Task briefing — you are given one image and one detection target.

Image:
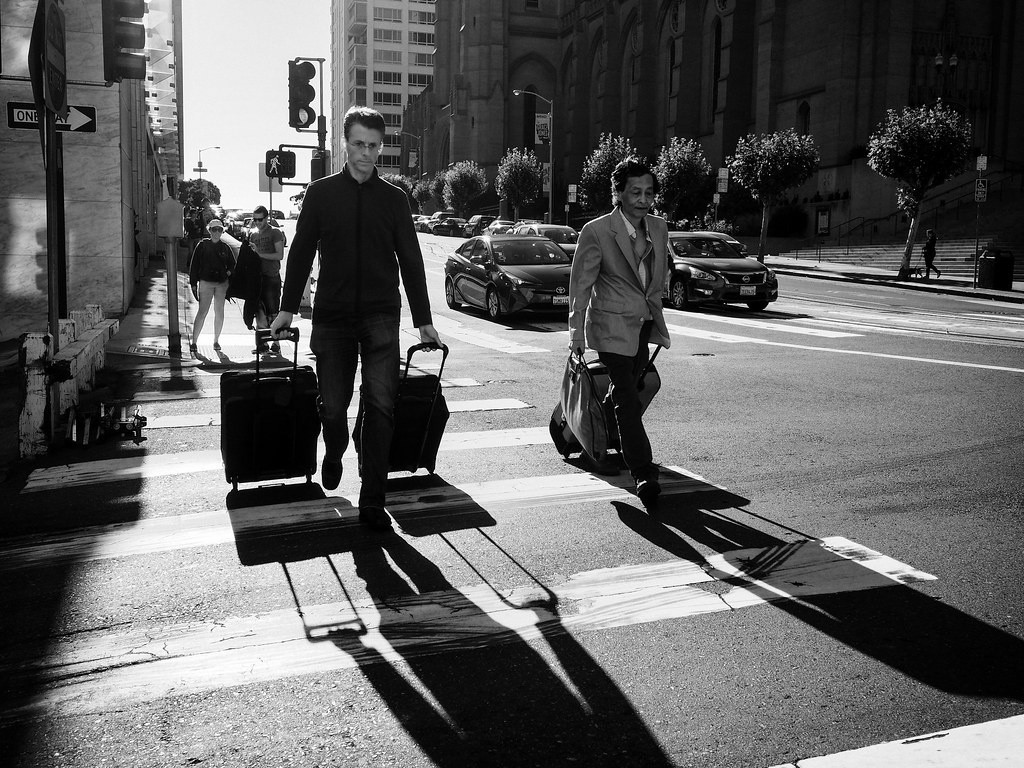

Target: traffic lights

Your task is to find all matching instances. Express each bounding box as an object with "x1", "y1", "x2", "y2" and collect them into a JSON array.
[
  {"x1": 101, "y1": 1, "x2": 147, "y2": 84},
  {"x1": 288, "y1": 60, "x2": 316, "y2": 129},
  {"x1": 265, "y1": 149, "x2": 296, "y2": 178}
]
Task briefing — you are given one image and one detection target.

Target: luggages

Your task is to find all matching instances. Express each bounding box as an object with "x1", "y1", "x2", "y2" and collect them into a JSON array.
[
  {"x1": 352, "y1": 341, "x2": 450, "y2": 474},
  {"x1": 550, "y1": 341, "x2": 662, "y2": 462},
  {"x1": 221, "y1": 327, "x2": 321, "y2": 490}
]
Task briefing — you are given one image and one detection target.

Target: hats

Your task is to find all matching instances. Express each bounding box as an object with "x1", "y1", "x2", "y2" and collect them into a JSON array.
[{"x1": 206, "y1": 219, "x2": 224, "y2": 234}]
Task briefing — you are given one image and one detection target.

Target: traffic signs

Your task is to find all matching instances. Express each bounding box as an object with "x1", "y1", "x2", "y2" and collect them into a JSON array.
[{"x1": 6, "y1": 101, "x2": 99, "y2": 133}]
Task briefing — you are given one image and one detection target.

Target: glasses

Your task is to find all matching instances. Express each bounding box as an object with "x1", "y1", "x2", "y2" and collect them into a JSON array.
[
  {"x1": 208, "y1": 227, "x2": 223, "y2": 232},
  {"x1": 253, "y1": 215, "x2": 266, "y2": 223},
  {"x1": 347, "y1": 141, "x2": 382, "y2": 153}
]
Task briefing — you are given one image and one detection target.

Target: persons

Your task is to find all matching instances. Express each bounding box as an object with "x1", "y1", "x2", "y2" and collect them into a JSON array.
[
  {"x1": 921, "y1": 229, "x2": 941, "y2": 279},
  {"x1": 189, "y1": 219, "x2": 236, "y2": 352},
  {"x1": 569, "y1": 161, "x2": 670, "y2": 498},
  {"x1": 270, "y1": 107, "x2": 444, "y2": 529},
  {"x1": 248, "y1": 206, "x2": 284, "y2": 354}
]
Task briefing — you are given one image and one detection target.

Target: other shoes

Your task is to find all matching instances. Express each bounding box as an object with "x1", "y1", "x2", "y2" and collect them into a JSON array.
[
  {"x1": 322, "y1": 452, "x2": 343, "y2": 490},
  {"x1": 923, "y1": 276, "x2": 929, "y2": 279},
  {"x1": 579, "y1": 451, "x2": 620, "y2": 475},
  {"x1": 635, "y1": 475, "x2": 662, "y2": 498},
  {"x1": 937, "y1": 270, "x2": 941, "y2": 278},
  {"x1": 269, "y1": 343, "x2": 281, "y2": 354},
  {"x1": 359, "y1": 507, "x2": 392, "y2": 527},
  {"x1": 213, "y1": 342, "x2": 220, "y2": 350},
  {"x1": 251, "y1": 344, "x2": 268, "y2": 354},
  {"x1": 190, "y1": 343, "x2": 197, "y2": 351}
]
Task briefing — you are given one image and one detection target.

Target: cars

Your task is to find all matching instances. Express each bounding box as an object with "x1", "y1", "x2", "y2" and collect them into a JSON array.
[
  {"x1": 211, "y1": 204, "x2": 318, "y2": 308},
  {"x1": 512, "y1": 224, "x2": 580, "y2": 261},
  {"x1": 444, "y1": 233, "x2": 573, "y2": 323},
  {"x1": 667, "y1": 231, "x2": 778, "y2": 313},
  {"x1": 412, "y1": 211, "x2": 544, "y2": 239}
]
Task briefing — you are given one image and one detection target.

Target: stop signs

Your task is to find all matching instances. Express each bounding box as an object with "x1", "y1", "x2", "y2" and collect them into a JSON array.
[{"x1": 45, "y1": 4, "x2": 67, "y2": 113}]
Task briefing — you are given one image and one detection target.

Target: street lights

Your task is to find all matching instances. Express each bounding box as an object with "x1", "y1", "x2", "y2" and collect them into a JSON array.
[
  {"x1": 199, "y1": 146, "x2": 221, "y2": 179},
  {"x1": 513, "y1": 90, "x2": 552, "y2": 225},
  {"x1": 394, "y1": 131, "x2": 422, "y2": 183}
]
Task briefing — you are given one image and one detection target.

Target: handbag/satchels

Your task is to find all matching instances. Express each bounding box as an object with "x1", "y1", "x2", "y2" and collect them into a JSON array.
[{"x1": 560, "y1": 342, "x2": 608, "y2": 462}]
